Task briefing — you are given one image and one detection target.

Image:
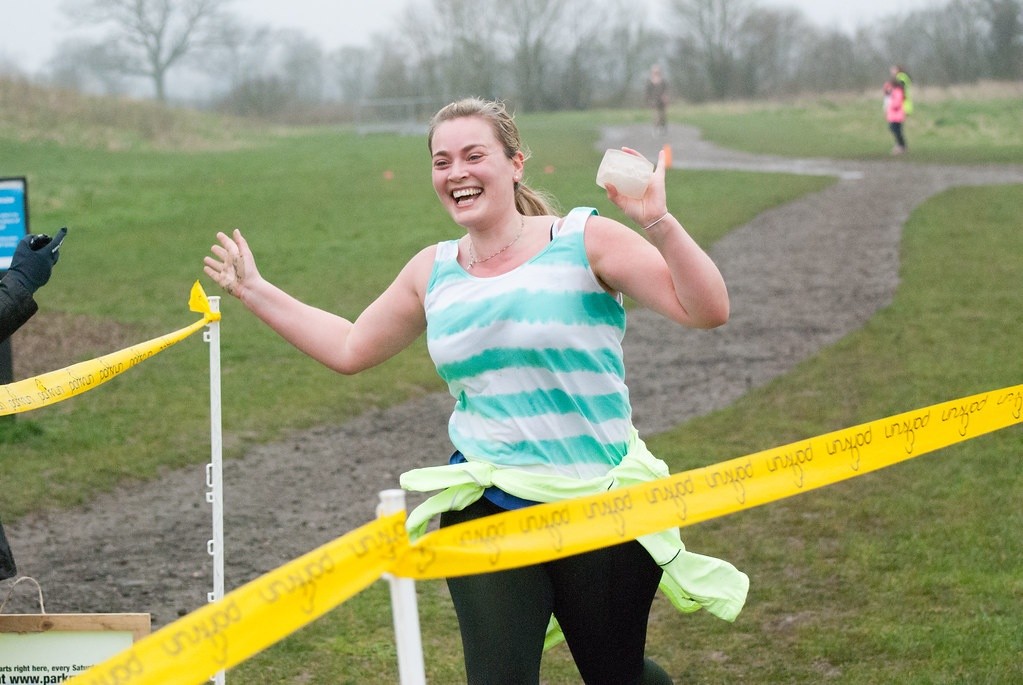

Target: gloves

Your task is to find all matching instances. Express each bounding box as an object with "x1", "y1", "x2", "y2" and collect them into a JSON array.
[{"x1": 5, "y1": 227, "x2": 68, "y2": 295}]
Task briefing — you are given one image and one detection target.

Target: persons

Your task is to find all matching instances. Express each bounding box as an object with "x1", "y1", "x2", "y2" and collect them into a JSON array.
[
  {"x1": 884, "y1": 64, "x2": 913, "y2": 156},
  {"x1": 645, "y1": 62, "x2": 669, "y2": 127},
  {"x1": 202, "y1": 94, "x2": 730, "y2": 685},
  {"x1": 0, "y1": 228, "x2": 68, "y2": 585}
]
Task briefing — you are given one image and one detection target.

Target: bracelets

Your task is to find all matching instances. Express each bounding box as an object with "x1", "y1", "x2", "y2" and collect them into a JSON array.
[{"x1": 643, "y1": 212, "x2": 669, "y2": 234}]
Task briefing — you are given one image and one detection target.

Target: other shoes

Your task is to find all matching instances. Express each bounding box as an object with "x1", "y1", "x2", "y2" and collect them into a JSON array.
[{"x1": 891, "y1": 146, "x2": 904, "y2": 156}]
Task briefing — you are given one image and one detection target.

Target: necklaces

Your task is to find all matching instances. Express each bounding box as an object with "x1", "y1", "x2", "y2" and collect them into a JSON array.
[{"x1": 467, "y1": 214, "x2": 526, "y2": 271}]
